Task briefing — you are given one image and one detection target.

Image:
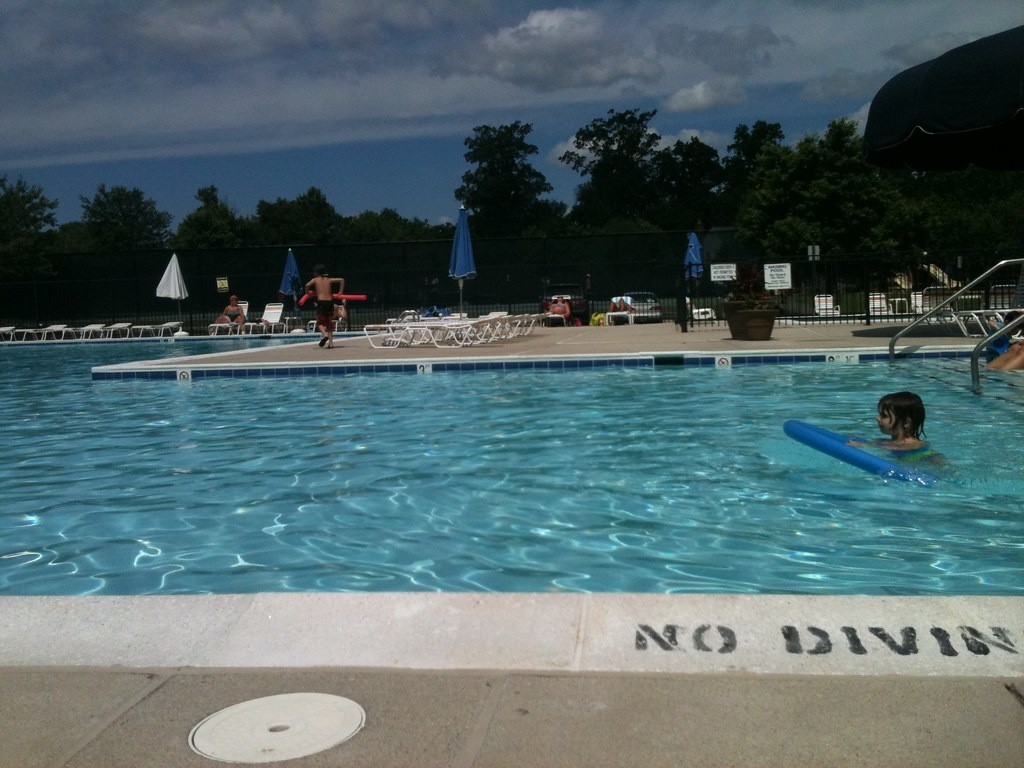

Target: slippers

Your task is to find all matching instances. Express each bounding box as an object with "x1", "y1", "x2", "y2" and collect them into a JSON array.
[
  {"x1": 327, "y1": 344, "x2": 334, "y2": 348},
  {"x1": 319, "y1": 337, "x2": 329, "y2": 347}
]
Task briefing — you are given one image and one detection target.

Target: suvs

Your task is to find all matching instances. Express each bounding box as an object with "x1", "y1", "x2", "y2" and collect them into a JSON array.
[{"x1": 541, "y1": 279, "x2": 591, "y2": 326}]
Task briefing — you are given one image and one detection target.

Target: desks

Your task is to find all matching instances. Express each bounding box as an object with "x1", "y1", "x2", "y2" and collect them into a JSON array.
[{"x1": 284, "y1": 317, "x2": 304, "y2": 333}]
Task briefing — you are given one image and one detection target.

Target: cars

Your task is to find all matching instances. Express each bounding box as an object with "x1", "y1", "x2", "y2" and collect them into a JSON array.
[{"x1": 619, "y1": 291, "x2": 665, "y2": 322}]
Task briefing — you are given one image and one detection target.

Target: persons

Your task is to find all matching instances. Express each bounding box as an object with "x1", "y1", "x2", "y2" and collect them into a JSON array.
[
  {"x1": 544, "y1": 296, "x2": 571, "y2": 317},
  {"x1": 305, "y1": 264, "x2": 348, "y2": 349},
  {"x1": 847, "y1": 391, "x2": 948, "y2": 469},
  {"x1": 984, "y1": 310, "x2": 1024, "y2": 371},
  {"x1": 608, "y1": 296, "x2": 636, "y2": 312},
  {"x1": 215, "y1": 294, "x2": 245, "y2": 335}
]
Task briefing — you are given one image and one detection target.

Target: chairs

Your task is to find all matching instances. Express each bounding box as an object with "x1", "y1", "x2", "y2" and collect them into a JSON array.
[
  {"x1": 911, "y1": 286, "x2": 953, "y2": 314},
  {"x1": 605, "y1": 296, "x2": 634, "y2": 325},
  {"x1": 0, "y1": 322, "x2": 183, "y2": 342},
  {"x1": 307, "y1": 300, "x2": 347, "y2": 333},
  {"x1": 911, "y1": 308, "x2": 1024, "y2": 338},
  {"x1": 989, "y1": 284, "x2": 1017, "y2": 310},
  {"x1": 813, "y1": 294, "x2": 840, "y2": 316},
  {"x1": 869, "y1": 292, "x2": 892, "y2": 314},
  {"x1": 684, "y1": 297, "x2": 717, "y2": 320},
  {"x1": 242, "y1": 302, "x2": 286, "y2": 335},
  {"x1": 542, "y1": 295, "x2": 571, "y2": 327},
  {"x1": 209, "y1": 301, "x2": 248, "y2": 336},
  {"x1": 364, "y1": 311, "x2": 548, "y2": 349}
]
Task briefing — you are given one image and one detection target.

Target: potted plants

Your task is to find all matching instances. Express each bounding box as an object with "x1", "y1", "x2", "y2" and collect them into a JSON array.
[{"x1": 720, "y1": 267, "x2": 779, "y2": 340}]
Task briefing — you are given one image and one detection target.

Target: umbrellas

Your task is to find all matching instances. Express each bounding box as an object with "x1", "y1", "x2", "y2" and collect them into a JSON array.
[
  {"x1": 449, "y1": 206, "x2": 477, "y2": 320},
  {"x1": 684, "y1": 232, "x2": 703, "y2": 279},
  {"x1": 278, "y1": 248, "x2": 302, "y2": 316},
  {"x1": 156, "y1": 253, "x2": 188, "y2": 331}
]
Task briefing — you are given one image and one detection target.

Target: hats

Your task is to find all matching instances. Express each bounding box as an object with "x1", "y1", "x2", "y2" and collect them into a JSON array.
[{"x1": 230, "y1": 295, "x2": 238, "y2": 301}]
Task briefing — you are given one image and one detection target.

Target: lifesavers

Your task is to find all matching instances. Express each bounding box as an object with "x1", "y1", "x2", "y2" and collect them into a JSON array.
[
  {"x1": 298, "y1": 292, "x2": 367, "y2": 305},
  {"x1": 783, "y1": 420, "x2": 942, "y2": 488},
  {"x1": 550, "y1": 304, "x2": 566, "y2": 315}
]
